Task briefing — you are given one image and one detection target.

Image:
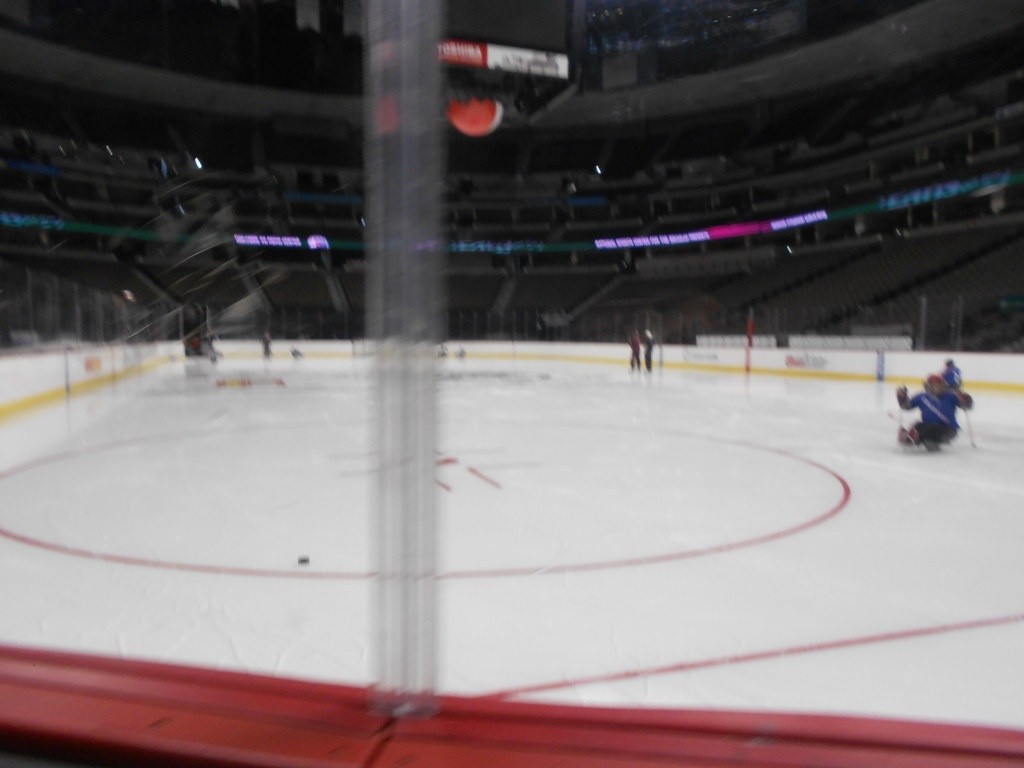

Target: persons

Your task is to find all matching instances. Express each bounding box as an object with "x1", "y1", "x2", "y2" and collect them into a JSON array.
[
  {"x1": 896, "y1": 359, "x2": 974, "y2": 452},
  {"x1": 627, "y1": 327, "x2": 656, "y2": 372}
]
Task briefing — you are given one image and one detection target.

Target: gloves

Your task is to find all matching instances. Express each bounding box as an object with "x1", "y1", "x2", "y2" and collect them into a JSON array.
[{"x1": 897, "y1": 388, "x2": 907, "y2": 403}]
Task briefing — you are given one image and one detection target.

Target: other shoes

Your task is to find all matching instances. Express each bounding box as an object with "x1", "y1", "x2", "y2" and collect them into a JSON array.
[{"x1": 899, "y1": 426, "x2": 920, "y2": 446}]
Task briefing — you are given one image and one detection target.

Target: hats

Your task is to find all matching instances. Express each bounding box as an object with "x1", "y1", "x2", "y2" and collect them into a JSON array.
[{"x1": 928, "y1": 375, "x2": 945, "y2": 384}]
